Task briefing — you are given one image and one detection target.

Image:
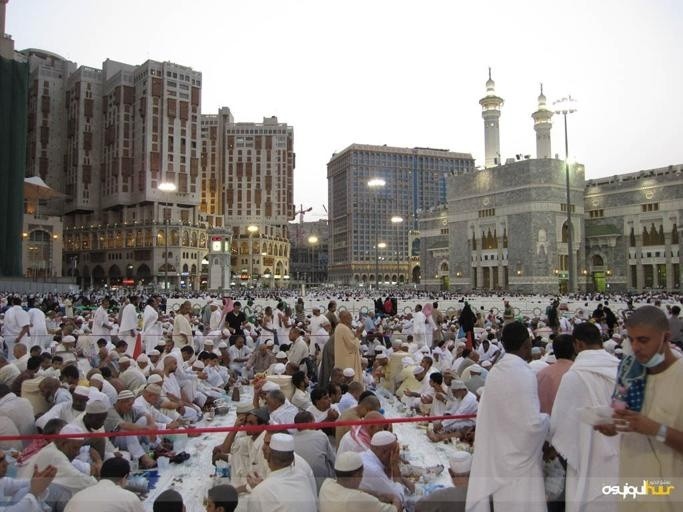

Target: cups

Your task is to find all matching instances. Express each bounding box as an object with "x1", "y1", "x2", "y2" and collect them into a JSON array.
[{"x1": 156, "y1": 456, "x2": 169, "y2": 478}]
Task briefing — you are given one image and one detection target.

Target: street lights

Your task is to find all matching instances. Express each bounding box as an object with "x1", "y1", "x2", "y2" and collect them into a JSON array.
[
  {"x1": 154, "y1": 180, "x2": 182, "y2": 295},
  {"x1": 245, "y1": 223, "x2": 258, "y2": 288},
  {"x1": 307, "y1": 237, "x2": 317, "y2": 286},
  {"x1": 365, "y1": 176, "x2": 388, "y2": 292},
  {"x1": 546, "y1": 94, "x2": 579, "y2": 297},
  {"x1": 389, "y1": 215, "x2": 403, "y2": 289},
  {"x1": 375, "y1": 241, "x2": 386, "y2": 285}
]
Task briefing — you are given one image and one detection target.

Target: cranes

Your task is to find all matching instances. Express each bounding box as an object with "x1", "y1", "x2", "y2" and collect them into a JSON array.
[{"x1": 287, "y1": 202, "x2": 312, "y2": 246}]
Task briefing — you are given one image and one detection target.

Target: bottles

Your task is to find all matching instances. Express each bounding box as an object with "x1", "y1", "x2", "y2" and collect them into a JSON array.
[{"x1": 231, "y1": 386, "x2": 240, "y2": 401}]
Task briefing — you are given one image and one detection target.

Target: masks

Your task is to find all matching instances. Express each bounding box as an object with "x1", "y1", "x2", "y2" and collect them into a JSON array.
[{"x1": 639, "y1": 351, "x2": 665, "y2": 369}]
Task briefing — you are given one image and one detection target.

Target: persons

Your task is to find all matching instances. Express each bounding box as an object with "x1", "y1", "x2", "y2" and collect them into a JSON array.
[{"x1": 0, "y1": 277, "x2": 683, "y2": 512}]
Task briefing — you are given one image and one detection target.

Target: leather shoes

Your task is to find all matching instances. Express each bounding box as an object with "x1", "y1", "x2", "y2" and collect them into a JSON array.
[{"x1": 170, "y1": 451, "x2": 190, "y2": 464}]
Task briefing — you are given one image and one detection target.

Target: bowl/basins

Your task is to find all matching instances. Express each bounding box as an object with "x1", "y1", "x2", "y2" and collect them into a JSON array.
[{"x1": 215, "y1": 406, "x2": 228, "y2": 414}]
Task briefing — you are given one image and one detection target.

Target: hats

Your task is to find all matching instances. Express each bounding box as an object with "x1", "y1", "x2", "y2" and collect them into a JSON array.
[
  {"x1": 333, "y1": 451, "x2": 364, "y2": 473},
  {"x1": 268, "y1": 433, "x2": 295, "y2": 453},
  {"x1": 342, "y1": 367, "x2": 357, "y2": 377},
  {"x1": 57, "y1": 332, "x2": 228, "y2": 443},
  {"x1": 360, "y1": 339, "x2": 491, "y2": 390},
  {"x1": 449, "y1": 451, "x2": 473, "y2": 474},
  {"x1": 262, "y1": 340, "x2": 288, "y2": 394},
  {"x1": 370, "y1": 429, "x2": 397, "y2": 448},
  {"x1": 236, "y1": 401, "x2": 271, "y2": 422}
]
